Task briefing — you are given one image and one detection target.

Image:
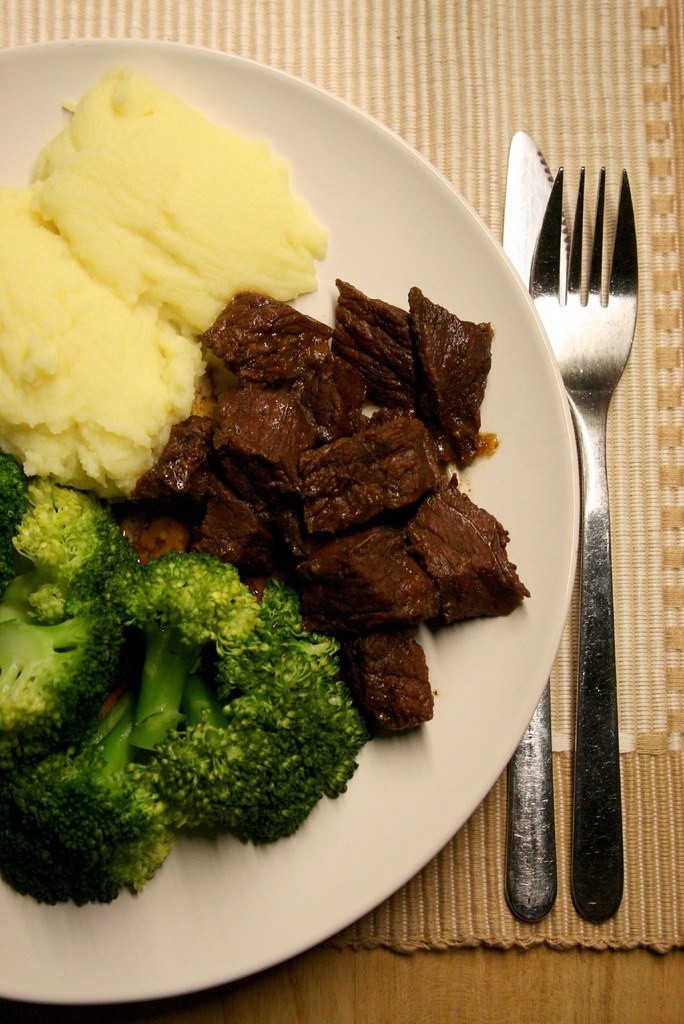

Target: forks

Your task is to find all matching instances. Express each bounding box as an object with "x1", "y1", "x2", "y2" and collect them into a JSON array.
[{"x1": 526, "y1": 165, "x2": 640, "y2": 914}]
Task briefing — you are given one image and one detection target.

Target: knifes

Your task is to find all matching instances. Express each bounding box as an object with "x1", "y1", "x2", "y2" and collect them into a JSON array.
[{"x1": 497, "y1": 131, "x2": 567, "y2": 922}]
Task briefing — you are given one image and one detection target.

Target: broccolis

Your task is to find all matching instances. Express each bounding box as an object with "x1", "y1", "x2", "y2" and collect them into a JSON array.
[{"x1": 0, "y1": 452, "x2": 371, "y2": 907}]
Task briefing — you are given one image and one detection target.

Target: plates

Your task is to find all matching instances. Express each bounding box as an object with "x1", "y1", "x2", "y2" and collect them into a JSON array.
[{"x1": 0, "y1": 38, "x2": 583, "y2": 1005}]
